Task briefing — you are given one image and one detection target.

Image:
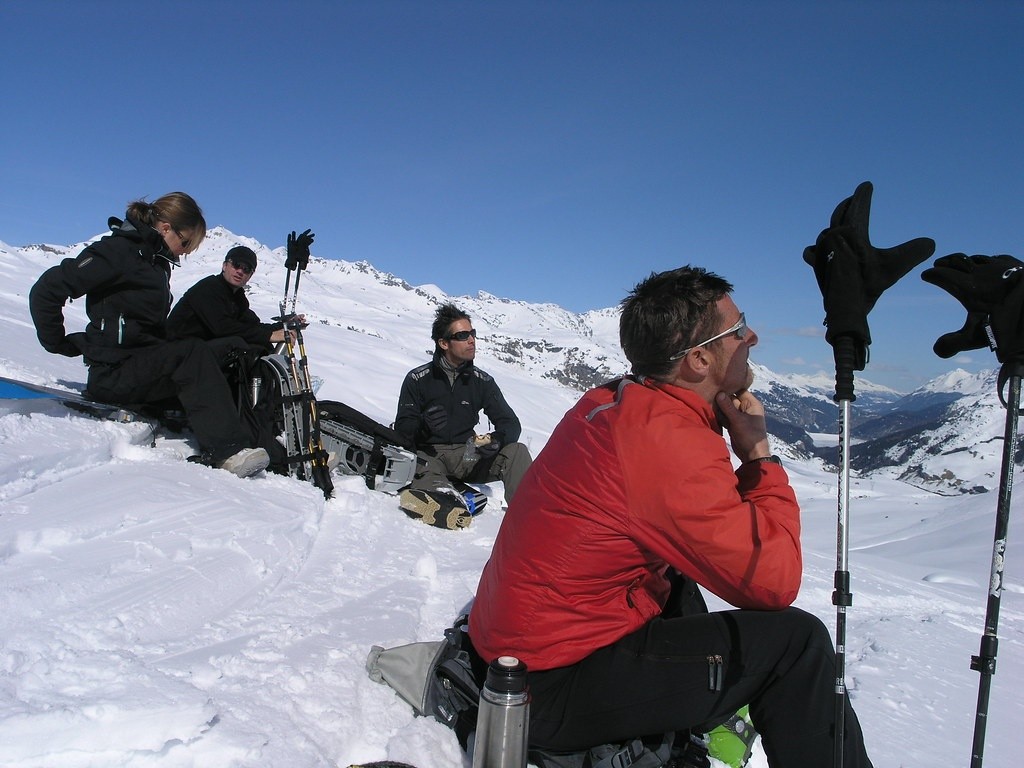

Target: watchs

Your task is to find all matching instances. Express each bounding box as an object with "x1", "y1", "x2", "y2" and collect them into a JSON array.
[{"x1": 740, "y1": 454, "x2": 783, "y2": 468}]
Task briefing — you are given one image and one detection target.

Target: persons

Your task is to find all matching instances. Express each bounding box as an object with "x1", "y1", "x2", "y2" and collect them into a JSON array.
[
  {"x1": 396, "y1": 303, "x2": 533, "y2": 530},
  {"x1": 29, "y1": 192, "x2": 270, "y2": 479},
  {"x1": 467, "y1": 265, "x2": 874, "y2": 768},
  {"x1": 169, "y1": 246, "x2": 306, "y2": 368}
]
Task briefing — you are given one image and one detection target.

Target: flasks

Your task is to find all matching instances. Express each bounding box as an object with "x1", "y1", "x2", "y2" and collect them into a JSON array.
[
  {"x1": 273, "y1": 319, "x2": 301, "y2": 357},
  {"x1": 249, "y1": 376, "x2": 263, "y2": 410},
  {"x1": 470, "y1": 657, "x2": 533, "y2": 768}
]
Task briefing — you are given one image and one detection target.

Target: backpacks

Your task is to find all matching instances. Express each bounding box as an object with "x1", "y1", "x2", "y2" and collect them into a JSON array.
[{"x1": 425, "y1": 595, "x2": 713, "y2": 768}]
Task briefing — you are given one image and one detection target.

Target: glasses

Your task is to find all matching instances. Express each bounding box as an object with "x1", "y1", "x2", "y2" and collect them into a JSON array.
[
  {"x1": 227, "y1": 260, "x2": 252, "y2": 274},
  {"x1": 669, "y1": 311, "x2": 749, "y2": 362},
  {"x1": 172, "y1": 226, "x2": 195, "y2": 252},
  {"x1": 443, "y1": 329, "x2": 477, "y2": 340}
]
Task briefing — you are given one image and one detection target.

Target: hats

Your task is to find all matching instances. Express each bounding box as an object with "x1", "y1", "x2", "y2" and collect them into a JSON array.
[{"x1": 225, "y1": 246, "x2": 259, "y2": 272}]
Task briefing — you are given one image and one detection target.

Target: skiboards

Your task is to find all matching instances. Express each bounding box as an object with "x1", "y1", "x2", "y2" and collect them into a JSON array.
[{"x1": 261, "y1": 353, "x2": 317, "y2": 488}]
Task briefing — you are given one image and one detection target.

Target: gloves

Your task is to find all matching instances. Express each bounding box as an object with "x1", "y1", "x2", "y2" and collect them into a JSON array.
[
  {"x1": 55, "y1": 336, "x2": 83, "y2": 359},
  {"x1": 284, "y1": 230, "x2": 300, "y2": 270},
  {"x1": 801, "y1": 180, "x2": 940, "y2": 346},
  {"x1": 295, "y1": 228, "x2": 317, "y2": 269},
  {"x1": 918, "y1": 248, "x2": 1024, "y2": 369}
]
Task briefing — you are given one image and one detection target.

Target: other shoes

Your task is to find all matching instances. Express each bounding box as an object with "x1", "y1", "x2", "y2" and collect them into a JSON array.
[
  {"x1": 398, "y1": 488, "x2": 473, "y2": 530},
  {"x1": 212, "y1": 447, "x2": 270, "y2": 478}
]
